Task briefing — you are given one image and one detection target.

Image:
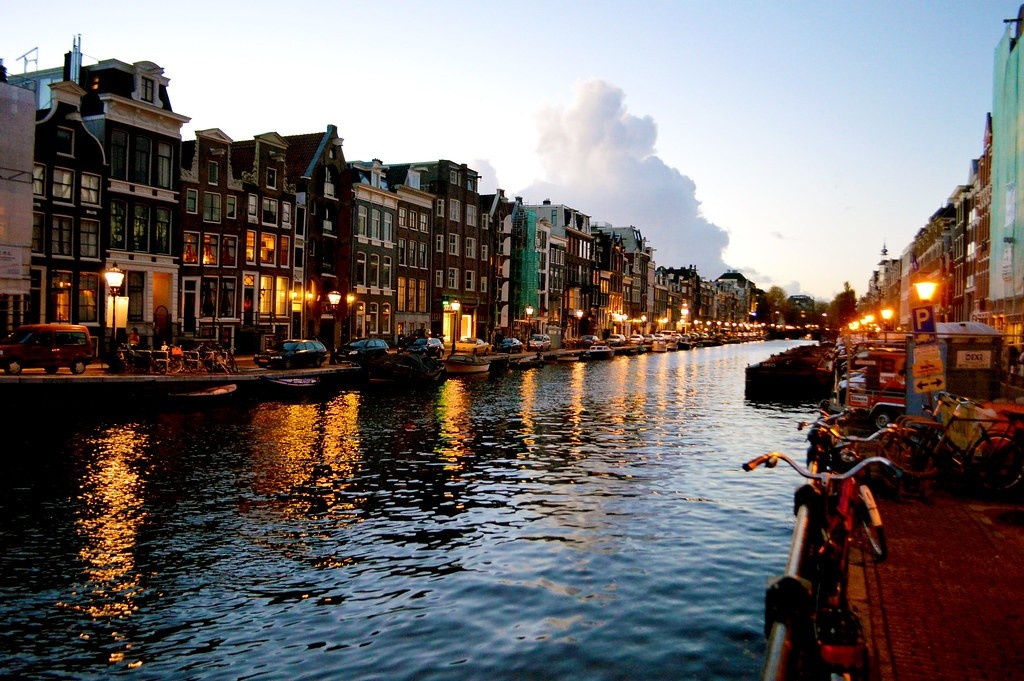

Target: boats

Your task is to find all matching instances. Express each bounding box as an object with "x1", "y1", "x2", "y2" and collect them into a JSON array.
[
  {"x1": 167, "y1": 385, "x2": 238, "y2": 396},
  {"x1": 270, "y1": 339, "x2": 768, "y2": 386}
]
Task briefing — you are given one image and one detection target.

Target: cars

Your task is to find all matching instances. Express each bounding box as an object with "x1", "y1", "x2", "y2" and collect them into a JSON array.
[
  {"x1": 579, "y1": 330, "x2": 711, "y2": 342},
  {"x1": 836, "y1": 336, "x2": 907, "y2": 407},
  {"x1": 499, "y1": 337, "x2": 523, "y2": 354},
  {"x1": 335, "y1": 338, "x2": 389, "y2": 365},
  {"x1": 397, "y1": 337, "x2": 445, "y2": 359},
  {"x1": 450, "y1": 337, "x2": 490, "y2": 356}
]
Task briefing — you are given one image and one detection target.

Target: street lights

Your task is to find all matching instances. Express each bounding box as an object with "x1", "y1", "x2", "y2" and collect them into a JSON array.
[
  {"x1": 105, "y1": 259, "x2": 126, "y2": 342},
  {"x1": 450, "y1": 300, "x2": 461, "y2": 354},
  {"x1": 526, "y1": 307, "x2": 533, "y2": 351},
  {"x1": 328, "y1": 290, "x2": 342, "y2": 366},
  {"x1": 848, "y1": 307, "x2": 894, "y2": 344},
  {"x1": 576, "y1": 310, "x2": 766, "y2": 338}
]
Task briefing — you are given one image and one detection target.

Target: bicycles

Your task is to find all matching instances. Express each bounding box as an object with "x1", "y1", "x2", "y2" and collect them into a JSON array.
[
  {"x1": 799, "y1": 393, "x2": 1024, "y2": 560},
  {"x1": 742, "y1": 446, "x2": 906, "y2": 681},
  {"x1": 120, "y1": 342, "x2": 239, "y2": 376}
]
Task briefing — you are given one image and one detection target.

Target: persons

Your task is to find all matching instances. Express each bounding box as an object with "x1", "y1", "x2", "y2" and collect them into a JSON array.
[
  {"x1": 128, "y1": 327, "x2": 140, "y2": 350},
  {"x1": 1005, "y1": 341, "x2": 1017, "y2": 374},
  {"x1": 161, "y1": 341, "x2": 168, "y2": 351},
  {"x1": 1017, "y1": 352, "x2": 1024, "y2": 377}
]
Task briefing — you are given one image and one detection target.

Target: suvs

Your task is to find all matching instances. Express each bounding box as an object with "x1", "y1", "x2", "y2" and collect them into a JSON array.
[
  {"x1": 529, "y1": 333, "x2": 553, "y2": 352},
  {"x1": 253, "y1": 340, "x2": 327, "y2": 371},
  {"x1": 0, "y1": 322, "x2": 94, "y2": 376}
]
